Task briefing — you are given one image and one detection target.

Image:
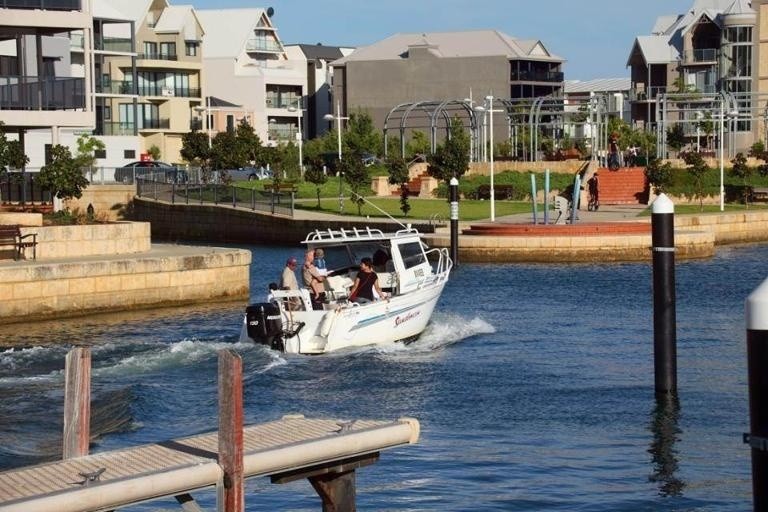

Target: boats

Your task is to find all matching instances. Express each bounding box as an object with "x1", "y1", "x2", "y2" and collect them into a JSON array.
[{"x1": 238, "y1": 221, "x2": 453, "y2": 358}]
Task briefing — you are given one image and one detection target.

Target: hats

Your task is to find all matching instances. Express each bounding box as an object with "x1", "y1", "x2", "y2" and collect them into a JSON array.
[
  {"x1": 286, "y1": 258, "x2": 298, "y2": 266},
  {"x1": 315, "y1": 249, "x2": 324, "y2": 256}
]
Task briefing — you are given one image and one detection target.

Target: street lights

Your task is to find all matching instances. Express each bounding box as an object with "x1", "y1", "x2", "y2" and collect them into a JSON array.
[
  {"x1": 194, "y1": 95, "x2": 220, "y2": 148},
  {"x1": 323, "y1": 100, "x2": 351, "y2": 164},
  {"x1": 288, "y1": 99, "x2": 307, "y2": 168},
  {"x1": 719, "y1": 101, "x2": 739, "y2": 211},
  {"x1": 266, "y1": 113, "x2": 276, "y2": 146},
  {"x1": 613, "y1": 92, "x2": 624, "y2": 120},
  {"x1": 464, "y1": 88, "x2": 506, "y2": 221}
]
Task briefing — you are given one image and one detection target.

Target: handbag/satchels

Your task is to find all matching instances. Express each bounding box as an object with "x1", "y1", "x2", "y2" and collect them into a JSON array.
[{"x1": 349, "y1": 288, "x2": 359, "y2": 303}]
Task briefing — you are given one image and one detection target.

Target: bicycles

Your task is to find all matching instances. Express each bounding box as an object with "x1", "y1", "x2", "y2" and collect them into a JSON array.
[
  {"x1": 609, "y1": 152, "x2": 620, "y2": 171},
  {"x1": 588, "y1": 189, "x2": 599, "y2": 211}
]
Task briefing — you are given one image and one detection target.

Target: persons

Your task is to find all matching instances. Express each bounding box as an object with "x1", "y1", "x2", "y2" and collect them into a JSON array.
[
  {"x1": 347, "y1": 257, "x2": 385, "y2": 304},
  {"x1": 373, "y1": 249, "x2": 389, "y2": 272},
  {"x1": 629, "y1": 144, "x2": 637, "y2": 165},
  {"x1": 587, "y1": 172, "x2": 600, "y2": 210},
  {"x1": 268, "y1": 248, "x2": 328, "y2": 312},
  {"x1": 608, "y1": 131, "x2": 620, "y2": 169}
]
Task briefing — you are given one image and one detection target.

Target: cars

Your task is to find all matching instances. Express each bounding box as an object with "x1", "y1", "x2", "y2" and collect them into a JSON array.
[
  {"x1": 114, "y1": 160, "x2": 188, "y2": 187},
  {"x1": 219, "y1": 163, "x2": 271, "y2": 182}
]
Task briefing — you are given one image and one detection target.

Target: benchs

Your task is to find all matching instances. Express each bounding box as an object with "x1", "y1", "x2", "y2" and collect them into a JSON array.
[
  {"x1": 262, "y1": 183, "x2": 299, "y2": 196},
  {"x1": 750, "y1": 188, "x2": 767, "y2": 203},
  {"x1": 0, "y1": 224, "x2": 39, "y2": 262},
  {"x1": 472, "y1": 184, "x2": 514, "y2": 202}
]
